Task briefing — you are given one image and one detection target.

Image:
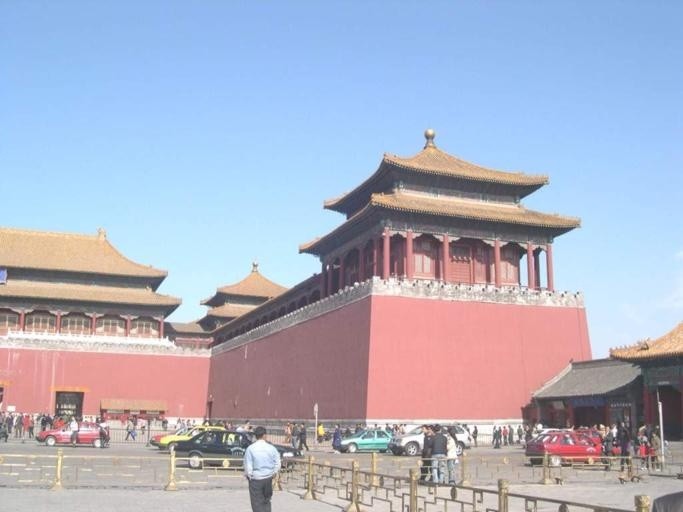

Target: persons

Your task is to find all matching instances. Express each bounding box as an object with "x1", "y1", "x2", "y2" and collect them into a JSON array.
[
  {"x1": 0, "y1": 409, "x2": 172, "y2": 449},
  {"x1": 461, "y1": 422, "x2": 545, "y2": 449},
  {"x1": 240, "y1": 424, "x2": 281, "y2": 512},
  {"x1": 284, "y1": 420, "x2": 408, "y2": 453},
  {"x1": 575, "y1": 421, "x2": 670, "y2": 473},
  {"x1": 419, "y1": 422, "x2": 460, "y2": 486},
  {"x1": 173, "y1": 417, "x2": 258, "y2": 444}
]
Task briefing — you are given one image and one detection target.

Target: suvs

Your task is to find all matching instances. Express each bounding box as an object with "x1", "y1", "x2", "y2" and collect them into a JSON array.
[{"x1": 389, "y1": 423, "x2": 475, "y2": 458}]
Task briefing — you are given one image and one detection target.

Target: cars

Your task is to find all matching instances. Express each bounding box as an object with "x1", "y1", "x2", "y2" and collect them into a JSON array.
[
  {"x1": 338, "y1": 428, "x2": 394, "y2": 452},
  {"x1": 150, "y1": 425, "x2": 298, "y2": 471},
  {"x1": 36, "y1": 421, "x2": 110, "y2": 447},
  {"x1": 524, "y1": 427, "x2": 656, "y2": 469}
]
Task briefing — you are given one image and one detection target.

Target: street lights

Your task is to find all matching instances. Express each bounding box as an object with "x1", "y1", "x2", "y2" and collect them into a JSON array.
[{"x1": 656, "y1": 380, "x2": 670, "y2": 472}]
